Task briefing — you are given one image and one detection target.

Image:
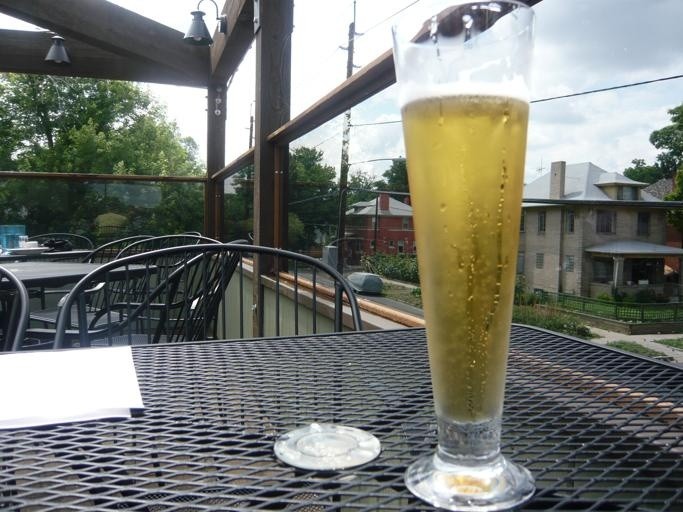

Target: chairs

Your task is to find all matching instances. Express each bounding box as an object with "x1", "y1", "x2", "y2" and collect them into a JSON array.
[{"x1": 0, "y1": 231, "x2": 362, "y2": 349}]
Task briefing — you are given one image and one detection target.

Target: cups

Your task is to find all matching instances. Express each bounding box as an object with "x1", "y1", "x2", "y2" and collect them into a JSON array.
[{"x1": 391, "y1": 0, "x2": 535, "y2": 512}]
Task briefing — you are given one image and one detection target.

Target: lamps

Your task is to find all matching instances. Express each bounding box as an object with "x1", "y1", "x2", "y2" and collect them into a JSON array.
[
  {"x1": 44, "y1": 35, "x2": 71, "y2": 65},
  {"x1": 183, "y1": 0, "x2": 226, "y2": 45}
]
